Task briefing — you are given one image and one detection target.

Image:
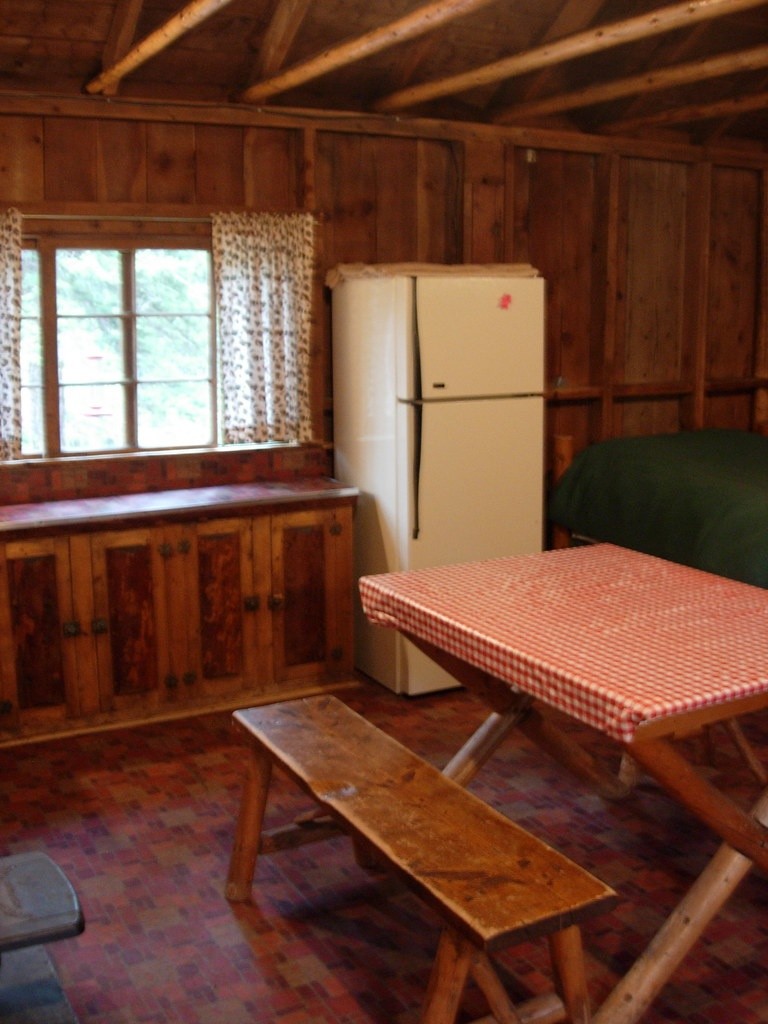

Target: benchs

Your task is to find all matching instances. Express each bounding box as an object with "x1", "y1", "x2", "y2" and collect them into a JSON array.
[{"x1": 229, "y1": 690, "x2": 619, "y2": 1024}]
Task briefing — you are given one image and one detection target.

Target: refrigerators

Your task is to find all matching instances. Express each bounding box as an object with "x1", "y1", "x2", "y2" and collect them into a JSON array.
[{"x1": 329, "y1": 263, "x2": 546, "y2": 699}]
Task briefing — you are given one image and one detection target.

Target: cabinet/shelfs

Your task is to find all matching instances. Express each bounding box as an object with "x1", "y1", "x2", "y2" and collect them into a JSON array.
[
  {"x1": 179, "y1": 505, "x2": 340, "y2": 698},
  {"x1": 0, "y1": 527, "x2": 172, "y2": 732}
]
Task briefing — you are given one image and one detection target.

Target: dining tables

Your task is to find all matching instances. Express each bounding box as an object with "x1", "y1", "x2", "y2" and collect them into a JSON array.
[{"x1": 359, "y1": 540, "x2": 766, "y2": 1024}]
